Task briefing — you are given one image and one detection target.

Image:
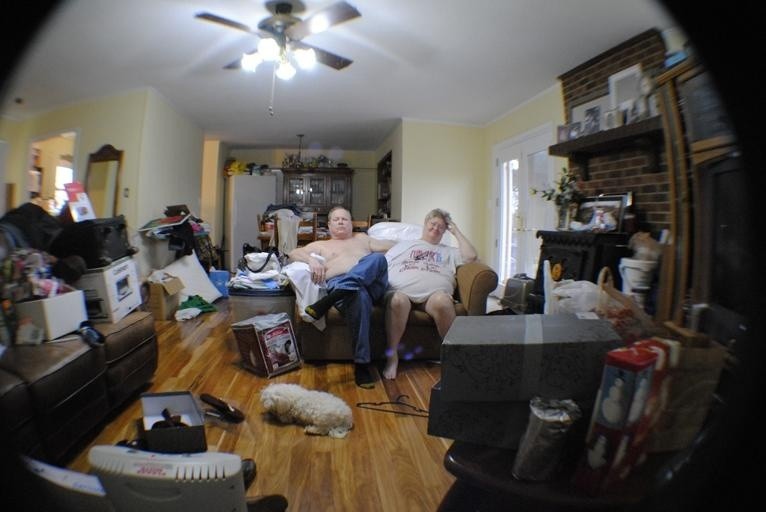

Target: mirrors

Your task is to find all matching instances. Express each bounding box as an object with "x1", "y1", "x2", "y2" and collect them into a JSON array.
[{"x1": 83, "y1": 144, "x2": 124, "y2": 223}]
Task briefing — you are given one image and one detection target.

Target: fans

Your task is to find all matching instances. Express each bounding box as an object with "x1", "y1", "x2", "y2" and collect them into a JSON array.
[{"x1": 195, "y1": 1, "x2": 359, "y2": 72}]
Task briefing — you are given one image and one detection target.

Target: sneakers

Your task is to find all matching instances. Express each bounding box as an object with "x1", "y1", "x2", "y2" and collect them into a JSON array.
[
  {"x1": 242, "y1": 457, "x2": 257, "y2": 490},
  {"x1": 245, "y1": 493, "x2": 288, "y2": 512}
]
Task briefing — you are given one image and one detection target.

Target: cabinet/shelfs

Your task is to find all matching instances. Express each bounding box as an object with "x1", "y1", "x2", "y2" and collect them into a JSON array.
[
  {"x1": 527, "y1": 232, "x2": 659, "y2": 315},
  {"x1": 328, "y1": 167, "x2": 352, "y2": 211},
  {"x1": 377, "y1": 149, "x2": 392, "y2": 218},
  {"x1": 286, "y1": 168, "x2": 328, "y2": 214}
]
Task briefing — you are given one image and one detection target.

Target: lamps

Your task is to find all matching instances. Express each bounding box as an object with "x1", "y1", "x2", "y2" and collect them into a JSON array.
[{"x1": 241, "y1": 36, "x2": 317, "y2": 115}]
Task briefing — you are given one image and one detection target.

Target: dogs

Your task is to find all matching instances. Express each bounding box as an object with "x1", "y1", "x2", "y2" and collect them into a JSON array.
[{"x1": 259, "y1": 382, "x2": 354, "y2": 437}]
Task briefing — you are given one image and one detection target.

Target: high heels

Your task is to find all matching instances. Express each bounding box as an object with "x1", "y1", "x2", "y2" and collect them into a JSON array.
[
  {"x1": 153, "y1": 407, "x2": 189, "y2": 430},
  {"x1": 200, "y1": 389, "x2": 245, "y2": 424}
]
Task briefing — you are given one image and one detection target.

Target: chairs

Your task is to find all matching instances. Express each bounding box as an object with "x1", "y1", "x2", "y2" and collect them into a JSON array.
[
  {"x1": 348, "y1": 213, "x2": 371, "y2": 237},
  {"x1": 257, "y1": 211, "x2": 317, "y2": 255},
  {"x1": 437, "y1": 301, "x2": 765, "y2": 512}
]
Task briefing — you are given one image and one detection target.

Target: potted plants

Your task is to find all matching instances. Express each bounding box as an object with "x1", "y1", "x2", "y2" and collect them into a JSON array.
[{"x1": 531, "y1": 167, "x2": 585, "y2": 233}]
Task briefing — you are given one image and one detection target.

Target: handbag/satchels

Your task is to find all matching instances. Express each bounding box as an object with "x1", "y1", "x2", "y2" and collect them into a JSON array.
[{"x1": 242, "y1": 242, "x2": 280, "y2": 273}]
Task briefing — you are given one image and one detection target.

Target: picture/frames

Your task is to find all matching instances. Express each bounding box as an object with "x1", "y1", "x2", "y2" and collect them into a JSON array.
[
  {"x1": 608, "y1": 62, "x2": 642, "y2": 112},
  {"x1": 570, "y1": 194, "x2": 626, "y2": 232}
]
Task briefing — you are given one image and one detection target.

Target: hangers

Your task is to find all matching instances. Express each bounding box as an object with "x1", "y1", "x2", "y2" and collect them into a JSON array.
[{"x1": 355, "y1": 395, "x2": 429, "y2": 418}]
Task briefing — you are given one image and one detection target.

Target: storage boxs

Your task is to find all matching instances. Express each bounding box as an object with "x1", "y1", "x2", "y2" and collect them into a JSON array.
[
  {"x1": 145, "y1": 276, "x2": 184, "y2": 320},
  {"x1": 440, "y1": 315, "x2": 623, "y2": 403},
  {"x1": 649, "y1": 336, "x2": 725, "y2": 456},
  {"x1": 15, "y1": 288, "x2": 87, "y2": 338},
  {"x1": 231, "y1": 314, "x2": 302, "y2": 379},
  {"x1": 426, "y1": 379, "x2": 591, "y2": 457},
  {"x1": 231, "y1": 286, "x2": 294, "y2": 325},
  {"x1": 80, "y1": 255, "x2": 142, "y2": 324}
]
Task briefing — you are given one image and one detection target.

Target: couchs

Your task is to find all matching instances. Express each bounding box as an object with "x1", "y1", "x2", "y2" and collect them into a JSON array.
[
  {"x1": 284, "y1": 241, "x2": 498, "y2": 364},
  {"x1": 1, "y1": 217, "x2": 159, "y2": 490}
]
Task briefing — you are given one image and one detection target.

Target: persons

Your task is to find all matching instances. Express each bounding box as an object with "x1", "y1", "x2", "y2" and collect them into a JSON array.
[
  {"x1": 290, "y1": 204, "x2": 394, "y2": 389},
  {"x1": 383, "y1": 207, "x2": 476, "y2": 380}
]
